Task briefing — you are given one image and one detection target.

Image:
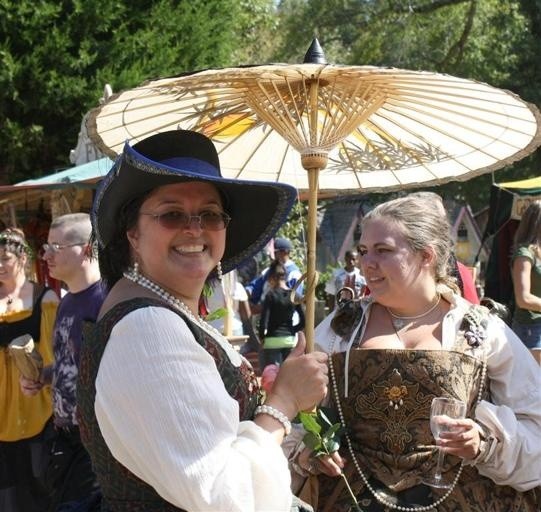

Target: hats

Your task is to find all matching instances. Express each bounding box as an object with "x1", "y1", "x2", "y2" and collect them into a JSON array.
[{"x1": 90, "y1": 131, "x2": 296, "y2": 278}]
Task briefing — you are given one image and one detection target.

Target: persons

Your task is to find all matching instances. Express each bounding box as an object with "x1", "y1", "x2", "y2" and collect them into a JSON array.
[
  {"x1": 22, "y1": 211, "x2": 107, "y2": 512},
  {"x1": 231, "y1": 235, "x2": 306, "y2": 376},
  {"x1": 74, "y1": 129, "x2": 328, "y2": 512},
  {"x1": 509, "y1": 200, "x2": 541, "y2": 361},
  {"x1": 283, "y1": 189, "x2": 541, "y2": 512},
  {"x1": 323, "y1": 248, "x2": 366, "y2": 315},
  {"x1": 1, "y1": 228, "x2": 62, "y2": 512}
]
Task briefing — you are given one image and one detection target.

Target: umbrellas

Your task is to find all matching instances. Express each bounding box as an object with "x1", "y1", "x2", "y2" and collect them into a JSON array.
[{"x1": 85, "y1": 39, "x2": 541, "y2": 355}]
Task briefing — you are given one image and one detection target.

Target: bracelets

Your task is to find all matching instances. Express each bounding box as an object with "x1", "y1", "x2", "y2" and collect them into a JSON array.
[{"x1": 253, "y1": 404, "x2": 293, "y2": 436}]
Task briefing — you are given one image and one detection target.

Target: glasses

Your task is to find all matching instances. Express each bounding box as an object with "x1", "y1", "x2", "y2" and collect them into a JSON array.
[
  {"x1": 41, "y1": 242, "x2": 84, "y2": 251},
  {"x1": 138, "y1": 209, "x2": 232, "y2": 230}
]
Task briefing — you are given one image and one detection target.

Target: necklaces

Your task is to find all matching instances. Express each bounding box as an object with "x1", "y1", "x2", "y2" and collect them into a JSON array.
[
  {"x1": 383, "y1": 292, "x2": 442, "y2": 320},
  {"x1": 117, "y1": 262, "x2": 245, "y2": 346}
]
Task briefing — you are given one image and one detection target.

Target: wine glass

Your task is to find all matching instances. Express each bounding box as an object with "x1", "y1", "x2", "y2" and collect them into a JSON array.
[{"x1": 418, "y1": 396, "x2": 467, "y2": 490}]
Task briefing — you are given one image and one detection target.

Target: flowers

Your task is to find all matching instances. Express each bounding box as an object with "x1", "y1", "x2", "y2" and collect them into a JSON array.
[{"x1": 261, "y1": 362, "x2": 364, "y2": 512}]
